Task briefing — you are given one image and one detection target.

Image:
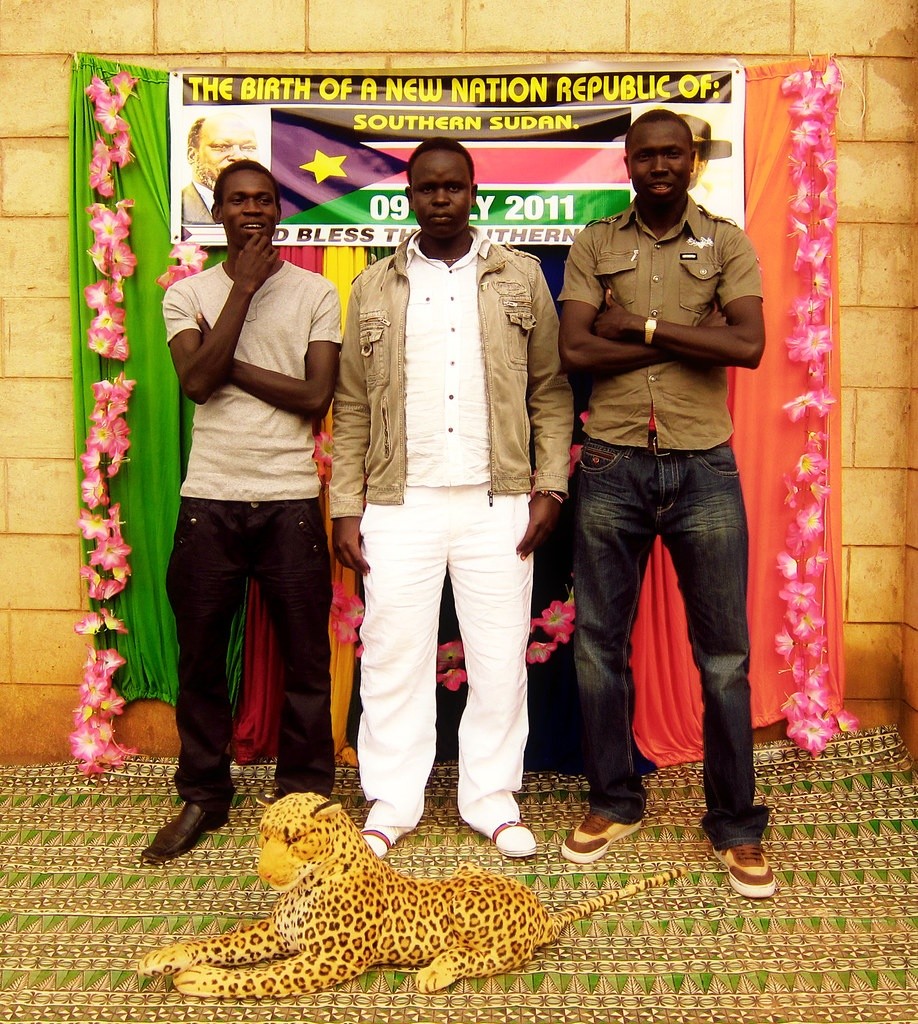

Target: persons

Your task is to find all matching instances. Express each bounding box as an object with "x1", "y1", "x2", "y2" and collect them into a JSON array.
[
  {"x1": 139, "y1": 160, "x2": 344, "y2": 860},
  {"x1": 556, "y1": 109, "x2": 775, "y2": 898},
  {"x1": 181, "y1": 113, "x2": 260, "y2": 225},
  {"x1": 663, "y1": 115, "x2": 711, "y2": 210},
  {"x1": 327, "y1": 136, "x2": 574, "y2": 861}
]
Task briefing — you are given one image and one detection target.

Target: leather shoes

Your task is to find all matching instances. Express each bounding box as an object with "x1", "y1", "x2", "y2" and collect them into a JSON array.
[{"x1": 141, "y1": 802, "x2": 226, "y2": 859}]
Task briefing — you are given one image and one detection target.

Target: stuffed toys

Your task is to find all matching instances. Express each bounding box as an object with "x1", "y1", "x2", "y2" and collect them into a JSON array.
[{"x1": 136, "y1": 789, "x2": 690, "y2": 1000}]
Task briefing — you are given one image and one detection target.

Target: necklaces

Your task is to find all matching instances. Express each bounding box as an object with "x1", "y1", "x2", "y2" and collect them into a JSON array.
[{"x1": 435, "y1": 257, "x2": 462, "y2": 263}]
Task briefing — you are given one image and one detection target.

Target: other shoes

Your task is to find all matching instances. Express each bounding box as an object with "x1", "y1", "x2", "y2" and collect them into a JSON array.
[
  {"x1": 360, "y1": 823, "x2": 413, "y2": 861},
  {"x1": 479, "y1": 820, "x2": 537, "y2": 858}
]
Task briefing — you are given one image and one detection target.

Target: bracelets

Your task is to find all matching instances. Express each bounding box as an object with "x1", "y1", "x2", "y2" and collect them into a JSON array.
[{"x1": 540, "y1": 489, "x2": 563, "y2": 506}]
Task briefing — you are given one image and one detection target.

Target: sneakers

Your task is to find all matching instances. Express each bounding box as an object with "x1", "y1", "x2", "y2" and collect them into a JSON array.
[
  {"x1": 560, "y1": 812, "x2": 642, "y2": 863},
  {"x1": 712, "y1": 841, "x2": 776, "y2": 898}
]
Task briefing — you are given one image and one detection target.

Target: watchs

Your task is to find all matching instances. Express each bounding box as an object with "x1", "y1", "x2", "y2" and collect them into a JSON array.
[{"x1": 644, "y1": 316, "x2": 658, "y2": 345}]
